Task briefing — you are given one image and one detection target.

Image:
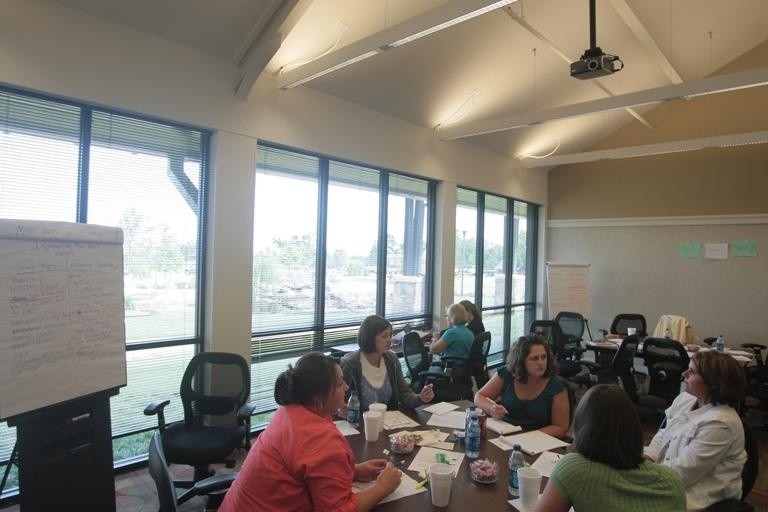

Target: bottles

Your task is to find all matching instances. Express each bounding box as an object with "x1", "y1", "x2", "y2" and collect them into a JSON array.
[
  {"x1": 463, "y1": 404, "x2": 479, "y2": 450},
  {"x1": 506, "y1": 444, "x2": 529, "y2": 498},
  {"x1": 465, "y1": 415, "x2": 480, "y2": 459},
  {"x1": 345, "y1": 390, "x2": 360, "y2": 428},
  {"x1": 715, "y1": 334, "x2": 724, "y2": 353},
  {"x1": 663, "y1": 328, "x2": 672, "y2": 340}
]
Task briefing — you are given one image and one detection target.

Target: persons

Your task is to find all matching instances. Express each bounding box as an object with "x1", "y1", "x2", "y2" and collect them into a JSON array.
[
  {"x1": 535, "y1": 384, "x2": 687, "y2": 512},
  {"x1": 473, "y1": 334, "x2": 570, "y2": 439},
  {"x1": 440, "y1": 300, "x2": 485, "y2": 337},
  {"x1": 331, "y1": 315, "x2": 435, "y2": 420},
  {"x1": 646, "y1": 349, "x2": 748, "y2": 512},
  {"x1": 428, "y1": 303, "x2": 475, "y2": 387},
  {"x1": 217, "y1": 351, "x2": 402, "y2": 512}
]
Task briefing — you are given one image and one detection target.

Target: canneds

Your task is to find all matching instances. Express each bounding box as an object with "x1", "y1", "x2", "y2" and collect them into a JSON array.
[{"x1": 476, "y1": 413, "x2": 487, "y2": 437}]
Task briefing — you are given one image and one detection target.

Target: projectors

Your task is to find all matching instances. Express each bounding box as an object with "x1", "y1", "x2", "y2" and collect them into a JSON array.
[{"x1": 571, "y1": 55, "x2": 615, "y2": 81}]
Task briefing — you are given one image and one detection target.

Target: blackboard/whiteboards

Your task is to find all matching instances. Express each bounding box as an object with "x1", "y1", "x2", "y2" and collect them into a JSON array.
[{"x1": 542, "y1": 220, "x2": 766, "y2": 365}]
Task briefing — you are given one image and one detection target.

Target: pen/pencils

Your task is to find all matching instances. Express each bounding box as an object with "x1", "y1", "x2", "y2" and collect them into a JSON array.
[
  {"x1": 375, "y1": 460, "x2": 406, "y2": 475},
  {"x1": 415, "y1": 477, "x2": 428, "y2": 490},
  {"x1": 486, "y1": 396, "x2": 510, "y2": 415}
]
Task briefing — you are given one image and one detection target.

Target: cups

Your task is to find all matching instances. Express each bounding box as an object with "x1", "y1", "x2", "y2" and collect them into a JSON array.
[
  {"x1": 628, "y1": 327, "x2": 637, "y2": 338},
  {"x1": 361, "y1": 410, "x2": 380, "y2": 443},
  {"x1": 515, "y1": 467, "x2": 542, "y2": 512},
  {"x1": 428, "y1": 463, "x2": 458, "y2": 509},
  {"x1": 368, "y1": 402, "x2": 387, "y2": 434},
  {"x1": 685, "y1": 344, "x2": 701, "y2": 353}
]
